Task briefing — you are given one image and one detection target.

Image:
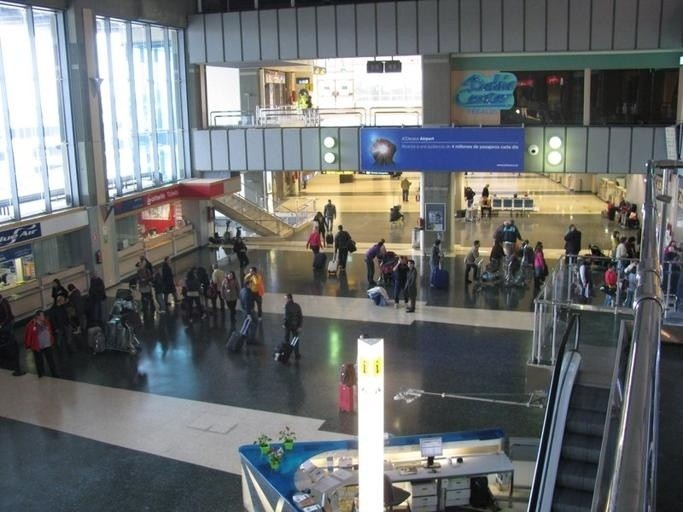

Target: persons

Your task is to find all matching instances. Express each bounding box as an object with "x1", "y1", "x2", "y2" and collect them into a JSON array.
[
  {"x1": 492, "y1": 219, "x2": 546, "y2": 290},
  {"x1": 604, "y1": 230, "x2": 640, "y2": 308},
  {"x1": 464, "y1": 186, "x2": 475, "y2": 206},
  {"x1": 322, "y1": 199, "x2": 336, "y2": 232},
  {"x1": 26, "y1": 271, "x2": 108, "y2": 378},
  {"x1": 663, "y1": 241, "x2": 683, "y2": 295},
  {"x1": 564, "y1": 224, "x2": 581, "y2": 264},
  {"x1": 305, "y1": 226, "x2": 323, "y2": 255},
  {"x1": 365, "y1": 239, "x2": 418, "y2": 312},
  {"x1": 297, "y1": 88, "x2": 312, "y2": 110},
  {"x1": 464, "y1": 240, "x2": 480, "y2": 284},
  {"x1": 577, "y1": 253, "x2": 592, "y2": 300},
  {"x1": 280, "y1": 294, "x2": 303, "y2": 360},
  {"x1": 466, "y1": 204, "x2": 478, "y2": 222},
  {"x1": 430, "y1": 239, "x2": 441, "y2": 288},
  {"x1": 619, "y1": 198, "x2": 633, "y2": 228},
  {"x1": 390, "y1": 204, "x2": 404, "y2": 222},
  {"x1": 480, "y1": 183, "x2": 489, "y2": 197},
  {"x1": 480, "y1": 196, "x2": 492, "y2": 217},
  {"x1": 332, "y1": 224, "x2": 351, "y2": 271},
  {"x1": 136, "y1": 254, "x2": 262, "y2": 322},
  {"x1": 233, "y1": 237, "x2": 249, "y2": 270},
  {"x1": 314, "y1": 211, "x2": 328, "y2": 247},
  {"x1": 399, "y1": 177, "x2": 410, "y2": 201}
]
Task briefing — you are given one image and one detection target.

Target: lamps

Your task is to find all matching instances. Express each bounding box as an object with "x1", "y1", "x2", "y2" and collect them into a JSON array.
[{"x1": 393, "y1": 386, "x2": 548, "y2": 410}]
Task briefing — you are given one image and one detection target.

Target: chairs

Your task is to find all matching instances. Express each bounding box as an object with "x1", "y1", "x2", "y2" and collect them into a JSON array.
[
  {"x1": 384, "y1": 473, "x2": 412, "y2": 511},
  {"x1": 490, "y1": 195, "x2": 536, "y2": 217}
]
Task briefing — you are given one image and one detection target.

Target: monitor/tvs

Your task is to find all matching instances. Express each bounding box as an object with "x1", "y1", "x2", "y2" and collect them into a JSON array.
[{"x1": 419, "y1": 437, "x2": 443, "y2": 468}]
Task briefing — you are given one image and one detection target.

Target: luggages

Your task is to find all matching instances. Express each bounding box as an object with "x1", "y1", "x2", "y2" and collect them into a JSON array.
[
  {"x1": 225, "y1": 318, "x2": 252, "y2": 354},
  {"x1": 313, "y1": 253, "x2": 326, "y2": 270},
  {"x1": 329, "y1": 252, "x2": 338, "y2": 275},
  {"x1": 272, "y1": 336, "x2": 300, "y2": 364},
  {"x1": 339, "y1": 382, "x2": 358, "y2": 414},
  {"x1": 88, "y1": 327, "x2": 104, "y2": 356},
  {"x1": 326, "y1": 232, "x2": 333, "y2": 244},
  {"x1": 432, "y1": 262, "x2": 449, "y2": 289}
]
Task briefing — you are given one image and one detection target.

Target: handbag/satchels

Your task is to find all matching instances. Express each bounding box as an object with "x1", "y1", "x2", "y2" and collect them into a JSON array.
[
  {"x1": 348, "y1": 239, "x2": 356, "y2": 252},
  {"x1": 207, "y1": 280, "x2": 218, "y2": 299},
  {"x1": 104, "y1": 289, "x2": 144, "y2": 357},
  {"x1": 338, "y1": 364, "x2": 355, "y2": 385}
]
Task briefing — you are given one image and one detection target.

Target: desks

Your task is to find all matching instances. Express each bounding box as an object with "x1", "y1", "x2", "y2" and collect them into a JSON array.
[
  {"x1": 481, "y1": 206, "x2": 491, "y2": 216},
  {"x1": 305, "y1": 466, "x2": 357, "y2": 512},
  {"x1": 384, "y1": 450, "x2": 515, "y2": 512}
]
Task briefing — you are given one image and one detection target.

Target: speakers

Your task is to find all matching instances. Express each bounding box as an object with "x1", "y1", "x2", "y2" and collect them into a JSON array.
[
  {"x1": 366, "y1": 62, "x2": 383, "y2": 72},
  {"x1": 385, "y1": 61, "x2": 400, "y2": 72}
]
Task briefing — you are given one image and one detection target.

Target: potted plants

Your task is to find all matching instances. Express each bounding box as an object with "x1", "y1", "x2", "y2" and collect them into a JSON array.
[{"x1": 252, "y1": 424, "x2": 297, "y2": 471}]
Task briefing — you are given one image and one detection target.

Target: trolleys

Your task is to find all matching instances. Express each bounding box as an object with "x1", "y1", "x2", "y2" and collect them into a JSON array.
[
  {"x1": 103, "y1": 314, "x2": 141, "y2": 356},
  {"x1": 472, "y1": 257, "x2": 501, "y2": 289}
]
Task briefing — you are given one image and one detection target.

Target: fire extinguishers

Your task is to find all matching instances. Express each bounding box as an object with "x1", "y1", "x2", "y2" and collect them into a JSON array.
[
  {"x1": 292, "y1": 171, "x2": 299, "y2": 183},
  {"x1": 96, "y1": 249, "x2": 102, "y2": 264},
  {"x1": 416, "y1": 218, "x2": 423, "y2": 230}
]
